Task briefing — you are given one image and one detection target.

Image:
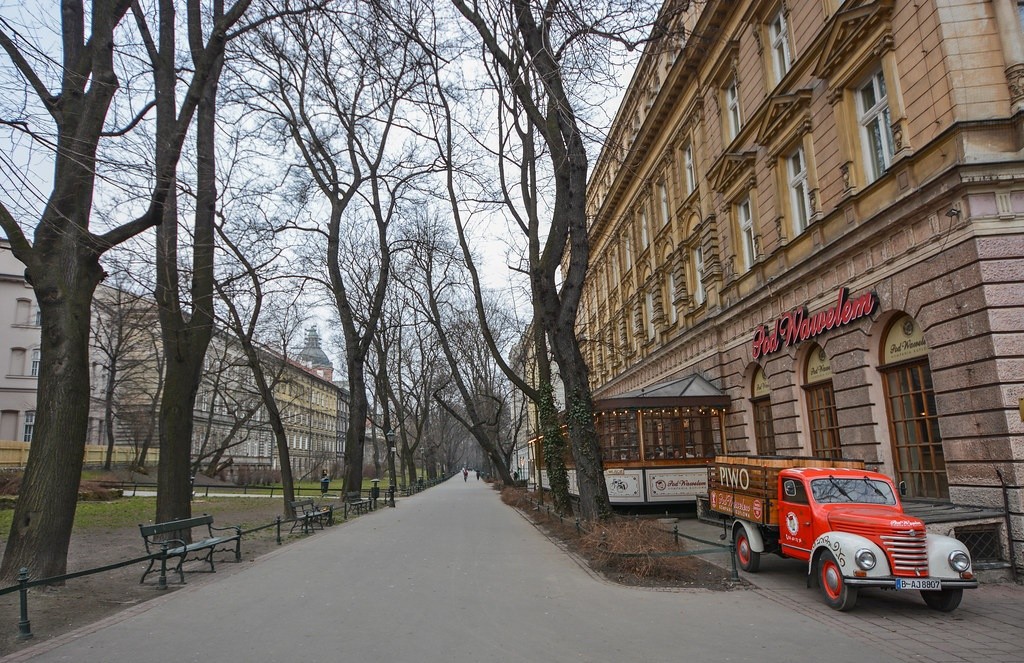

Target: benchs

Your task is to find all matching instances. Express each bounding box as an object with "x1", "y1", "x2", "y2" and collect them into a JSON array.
[
  {"x1": 343, "y1": 490, "x2": 374, "y2": 516},
  {"x1": 138, "y1": 512, "x2": 242, "y2": 586},
  {"x1": 287, "y1": 497, "x2": 336, "y2": 535}
]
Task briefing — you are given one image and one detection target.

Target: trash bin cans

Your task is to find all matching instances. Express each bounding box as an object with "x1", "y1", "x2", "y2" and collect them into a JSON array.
[{"x1": 321, "y1": 479, "x2": 330, "y2": 493}]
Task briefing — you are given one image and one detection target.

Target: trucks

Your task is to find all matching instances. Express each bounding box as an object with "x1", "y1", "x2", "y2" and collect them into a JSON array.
[{"x1": 706, "y1": 453, "x2": 980, "y2": 613}]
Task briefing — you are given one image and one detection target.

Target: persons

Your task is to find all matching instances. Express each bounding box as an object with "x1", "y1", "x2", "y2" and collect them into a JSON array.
[
  {"x1": 513, "y1": 471, "x2": 518, "y2": 482},
  {"x1": 464, "y1": 470, "x2": 468, "y2": 482},
  {"x1": 476, "y1": 468, "x2": 481, "y2": 480}
]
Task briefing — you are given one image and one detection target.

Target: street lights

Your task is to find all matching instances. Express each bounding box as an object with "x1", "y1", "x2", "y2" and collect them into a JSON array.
[
  {"x1": 386, "y1": 430, "x2": 395, "y2": 507},
  {"x1": 420, "y1": 446, "x2": 425, "y2": 476}
]
